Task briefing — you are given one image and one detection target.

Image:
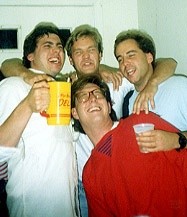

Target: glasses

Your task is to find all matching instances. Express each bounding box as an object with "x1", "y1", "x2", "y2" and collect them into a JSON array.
[{"x1": 74, "y1": 88, "x2": 106, "y2": 103}]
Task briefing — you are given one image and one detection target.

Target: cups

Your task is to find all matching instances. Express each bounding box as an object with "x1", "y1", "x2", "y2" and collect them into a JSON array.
[
  {"x1": 133, "y1": 123, "x2": 154, "y2": 153},
  {"x1": 40, "y1": 81, "x2": 71, "y2": 126}
]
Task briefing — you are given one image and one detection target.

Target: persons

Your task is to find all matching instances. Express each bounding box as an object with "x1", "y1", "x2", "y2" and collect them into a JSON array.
[{"x1": 0, "y1": 28, "x2": 187, "y2": 217}]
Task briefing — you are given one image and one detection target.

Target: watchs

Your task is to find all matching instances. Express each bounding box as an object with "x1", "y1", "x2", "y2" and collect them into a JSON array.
[{"x1": 174, "y1": 132, "x2": 187, "y2": 152}]
